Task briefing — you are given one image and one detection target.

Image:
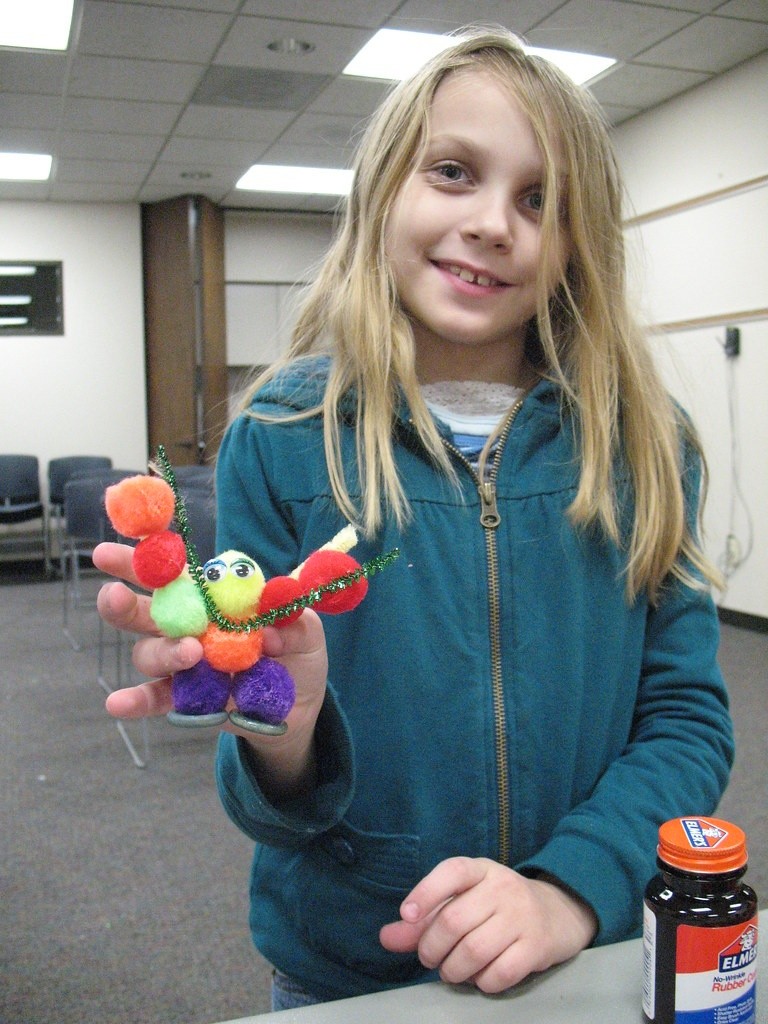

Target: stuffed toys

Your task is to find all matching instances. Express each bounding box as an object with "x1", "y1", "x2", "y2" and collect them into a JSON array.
[{"x1": 104, "y1": 475, "x2": 367, "y2": 738}]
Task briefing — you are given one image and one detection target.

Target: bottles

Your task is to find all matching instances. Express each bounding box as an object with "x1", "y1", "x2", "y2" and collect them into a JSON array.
[{"x1": 641, "y1": 814, "x2": 759, "y2": 1023}]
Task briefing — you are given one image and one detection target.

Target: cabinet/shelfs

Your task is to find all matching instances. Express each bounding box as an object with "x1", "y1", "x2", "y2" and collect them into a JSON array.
[
  {"x1": 595, "y1": 51, "x2": 768, "y2": 633},
  {"x1": 224, "y1": 214, "x2": 337, "y2": 366}
]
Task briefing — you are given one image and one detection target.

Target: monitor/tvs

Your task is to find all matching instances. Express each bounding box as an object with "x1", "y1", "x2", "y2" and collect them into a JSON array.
[{"x1": 0, "y1": 260, "x2": 64, "y2": 337}]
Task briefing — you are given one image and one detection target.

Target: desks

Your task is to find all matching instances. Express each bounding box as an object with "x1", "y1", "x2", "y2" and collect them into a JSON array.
[{"x1": 226, "y1": 911, "x2": 768, "y2": 1024}]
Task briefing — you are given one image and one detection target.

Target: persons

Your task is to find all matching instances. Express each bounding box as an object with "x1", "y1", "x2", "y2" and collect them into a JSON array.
[{"x1": 91, "y1": 23, "x2": 734, "y2": 1015}]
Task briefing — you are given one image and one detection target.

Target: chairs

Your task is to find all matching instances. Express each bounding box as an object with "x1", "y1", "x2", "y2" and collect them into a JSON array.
[{"x1": 0, "y1": 454, "x2": 214, "y2": 769}]
